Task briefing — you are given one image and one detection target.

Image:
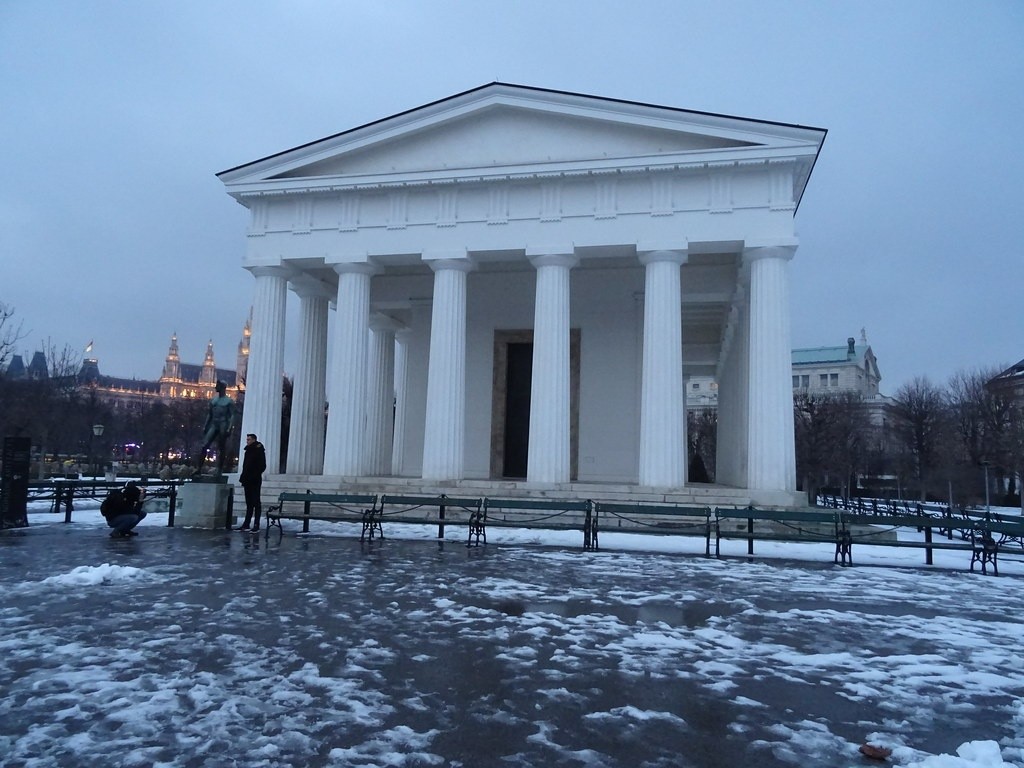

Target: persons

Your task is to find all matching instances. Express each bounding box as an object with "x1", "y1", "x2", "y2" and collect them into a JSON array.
[
  {"x1": 190, "y1": 379, "x2": 234, "y2": 478},
  {"x1": 100, "y1": 485, "x2": 147, "y2": 538},
  {"x1": 233, "y1": 434, "x2": 266, "y2": 534}
]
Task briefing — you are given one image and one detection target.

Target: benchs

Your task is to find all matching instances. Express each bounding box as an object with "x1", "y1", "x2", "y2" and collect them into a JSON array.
[{"x1": 265, "y1": 491, "x2": 1024, "y2": 576}]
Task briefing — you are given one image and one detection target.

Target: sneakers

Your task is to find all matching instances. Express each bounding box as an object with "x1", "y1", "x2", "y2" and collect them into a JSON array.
[
  {"x1": 109, "y1": 531, "x2": 140, "y2": 538},
  {"x1": 233, "y1": 526, "x2": 262, "y2": 534}
]
